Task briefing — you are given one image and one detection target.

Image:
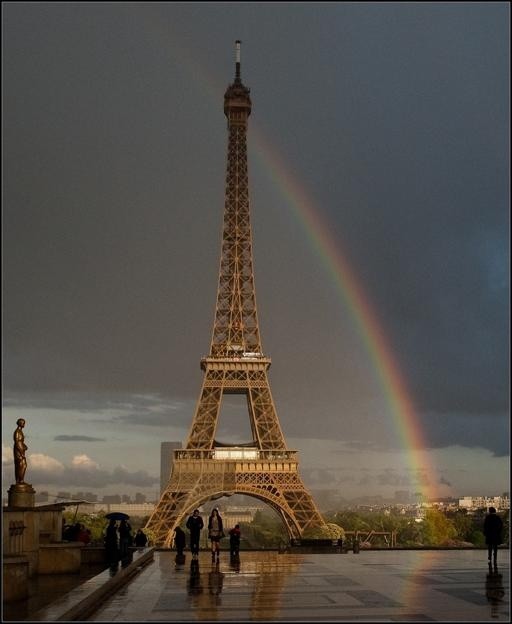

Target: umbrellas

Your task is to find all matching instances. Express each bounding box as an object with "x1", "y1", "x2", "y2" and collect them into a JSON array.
[{"x1": 104, "y1": 512, "x2": 130, "y2": 522}]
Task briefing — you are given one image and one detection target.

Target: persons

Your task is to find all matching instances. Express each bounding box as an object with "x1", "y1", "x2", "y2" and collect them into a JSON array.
[
  {"x1": 483, "y1": 506, "x2": 505, "y2": 565},
  {"x1": 228, "y1": 523, "x2": 243, "y2": 558},
  {"x1": 133, "y1": 527, "x2": 149, "y2": 548},
  {"x1": 207, "y1": 507, "x2": 228, "y2": 565},
  {"x1": 13, "y1": 417, "x2": 29, "y2": 483},
  {"x1": 63, "y1": 516, "x2": 93, "y2": 547},
  {"x1": 186, "y1": 509, "x2": 206, "y2": 566},
  {"x1": 207, "y1": 558, "x2": 225, "y2": 607},
  {"x1": 484, "y1": 561, "x2": 506, "y2": 620},
  {"x1": 186, "y1": 558, "x2": 205, "y2": 604},
  {"x1": 174, "y1": 526, "x2": 186, "y2": 557},
  {"x1": 105, "y1": 515, "x2": 118, "y2": 560},
  {"x1": 118, "y1": 518, "x2": 132, "y2": 560}
]
